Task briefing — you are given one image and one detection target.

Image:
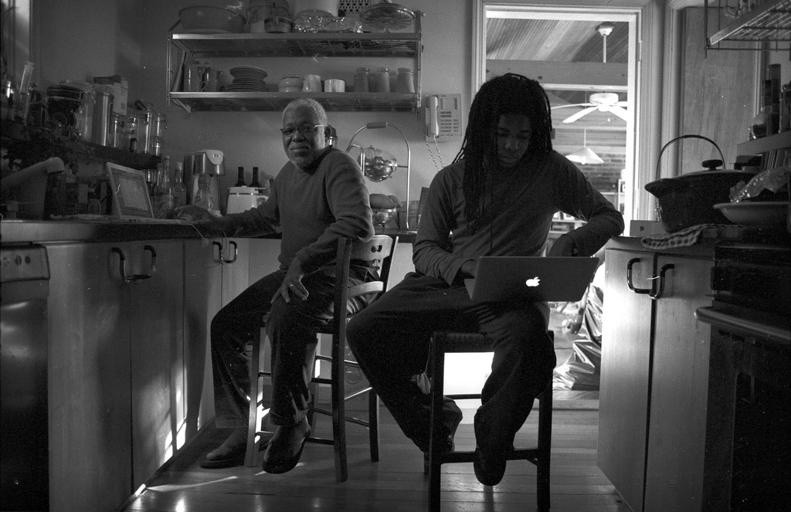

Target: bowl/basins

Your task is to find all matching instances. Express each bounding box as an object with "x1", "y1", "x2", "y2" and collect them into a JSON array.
[
  {"x1": 178, "y1": 6, "x2": 247, "y2": 34},
  {"x1": 713, "y1": 200, "x2": 790, "y2": 226}
]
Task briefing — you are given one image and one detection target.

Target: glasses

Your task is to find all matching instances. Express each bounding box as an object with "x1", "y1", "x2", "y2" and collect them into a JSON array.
[{"x1": 279, "y1": 124, "x2": 327, "y2": 135}]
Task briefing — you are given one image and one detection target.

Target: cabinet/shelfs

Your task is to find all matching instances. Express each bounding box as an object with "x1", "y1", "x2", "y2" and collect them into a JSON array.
[
  {"x1": 596, "y1": 234, "x2": 791, "y2": 512},
  {"x1": 178, "y1": 231, "x2": 249, "y2": 462},
  {"x1": 28, "y1": 232, "x2": 188, "y2": 512},
  {"x1": 159, "y1": 7, "x2": 431, "y2": 123}
]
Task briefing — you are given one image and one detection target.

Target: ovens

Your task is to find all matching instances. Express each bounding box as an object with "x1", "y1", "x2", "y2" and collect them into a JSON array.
[{"x1": 0, "y1": 242, "x2": 52, "y2": 512}]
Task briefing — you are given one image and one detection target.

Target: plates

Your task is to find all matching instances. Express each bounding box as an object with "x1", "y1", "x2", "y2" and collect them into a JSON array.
[{"x1": 227, "y1": 66, "x2": 269, "y2": 92}]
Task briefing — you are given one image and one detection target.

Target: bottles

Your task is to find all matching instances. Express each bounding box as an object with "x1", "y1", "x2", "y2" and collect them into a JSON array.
[
  {"x1": 373, "y1": 67, "x2": 391, "y2": 93},
  {"x1": 148, "y1": 154, "x2": 186, "y2": 220},
  {"x1": 250, "y1": 166, "x2": 263, "y2": 196},
  {"x1": 750, "y1": 79, "x2": 776, "y2": 139},
  {"x1": 396, "y1": 68, "x2": 416, "y2": 93},
  {"x1": 353, "y1": 67, "x2": 372, "y2": 92},
  {"x1": 234, "y1": 167, "x2": 247, "y2": 186},
  {"x1": 62, "y1": 78, "x2": 168, "y2": 156}
]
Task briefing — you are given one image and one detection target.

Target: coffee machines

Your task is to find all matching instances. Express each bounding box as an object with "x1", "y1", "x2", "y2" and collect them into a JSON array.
[{"x1": 184, "y1": 150, "x2": 226, "y2": 217}]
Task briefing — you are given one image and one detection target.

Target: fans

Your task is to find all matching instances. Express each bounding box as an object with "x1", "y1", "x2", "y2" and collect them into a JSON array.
[{"x1": 543, "y1": 22, "x2": 630, "y2": 128}]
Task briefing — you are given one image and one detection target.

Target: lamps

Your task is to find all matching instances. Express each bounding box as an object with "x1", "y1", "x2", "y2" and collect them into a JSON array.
[{"x1": 559, "y1": 87, "x2": 607, "y2": 168}]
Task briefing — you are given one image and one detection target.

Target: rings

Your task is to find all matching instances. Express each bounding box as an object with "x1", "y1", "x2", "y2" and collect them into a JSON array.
[{"x1": 289, "y1": 284, "x2": 295, "y2": 288}]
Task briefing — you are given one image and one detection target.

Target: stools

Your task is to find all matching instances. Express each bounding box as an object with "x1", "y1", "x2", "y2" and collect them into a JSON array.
[{"x1": 417, "y1": 324, "x2": 557, "y2": 510}]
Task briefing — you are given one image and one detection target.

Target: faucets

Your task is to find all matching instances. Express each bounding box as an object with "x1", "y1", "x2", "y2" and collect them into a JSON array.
[{"x1": 1, "y1": 155, "x2": 66, "y2": 194}]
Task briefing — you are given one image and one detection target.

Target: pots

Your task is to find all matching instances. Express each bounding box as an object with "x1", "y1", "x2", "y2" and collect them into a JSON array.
[
  {"x1": 358, "y1": 4, "x2": 418, "y2": 34},
  {"x1": 645, "y1": 159, "x2": 757, "y2": 234}
]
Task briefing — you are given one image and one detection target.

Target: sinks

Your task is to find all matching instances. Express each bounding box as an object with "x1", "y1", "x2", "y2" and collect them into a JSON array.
[{"x1": 0, "y1": 219, "x2": 99, "y2": 243}]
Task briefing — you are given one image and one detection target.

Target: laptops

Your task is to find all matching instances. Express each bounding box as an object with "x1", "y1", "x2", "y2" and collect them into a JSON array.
[
  {"x1": 464, "y1": 256, "x2": 600, "y2": 301},
  {"x1": 105, "y1": 161, "x2": 186, "y2": 225}
]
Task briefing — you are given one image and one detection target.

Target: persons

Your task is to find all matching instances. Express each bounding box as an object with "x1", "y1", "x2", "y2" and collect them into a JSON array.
[
  {"x1": 342, "y1": 71, "x2": 625, "y2": 487},
  {"x1": 173, "y1": 97, "x2": 382, "y2": 475}
]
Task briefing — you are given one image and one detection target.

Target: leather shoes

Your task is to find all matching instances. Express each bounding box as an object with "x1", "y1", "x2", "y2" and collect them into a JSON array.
[
  {"x1": 262, "y1": 416, "x2": 310, "y2": 474},
  {"x1": 199, "y1": 426, "x2": 268, "y2": 469},
  {"x1": 473, "y1": 439, "x2": 506, "y2": 486},
  {"x1": 423, "y1": 413, "x2": 464, "y2": 461}
]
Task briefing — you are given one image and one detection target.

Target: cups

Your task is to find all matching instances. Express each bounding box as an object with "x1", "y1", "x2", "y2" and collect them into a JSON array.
[
  {"x1": 263, "y1": 16, "x2": 293, "y2": 33},
  {"x1": 301, "y1": 75, "x2": 324, "y2": 93},
  {"x1": 325, "y1": 79, "x2": 346, "y2": 93},
  {"x1": 203, "y1": 69, "x2": 216, "y2": 91},
  {"x1": 183, "y1": 64, "x2": 207, "y2": 91},
  {"x1": 226, "y1": 186, "x2": 269, "y2": 214},
  {"x1": 279, "y1": 76, "x2": 301, "y2": 94}
]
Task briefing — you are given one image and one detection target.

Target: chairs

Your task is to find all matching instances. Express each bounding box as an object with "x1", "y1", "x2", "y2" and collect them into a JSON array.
[{"x1": 242, "y1": 230, "x2": 404, "y2": 484}]
